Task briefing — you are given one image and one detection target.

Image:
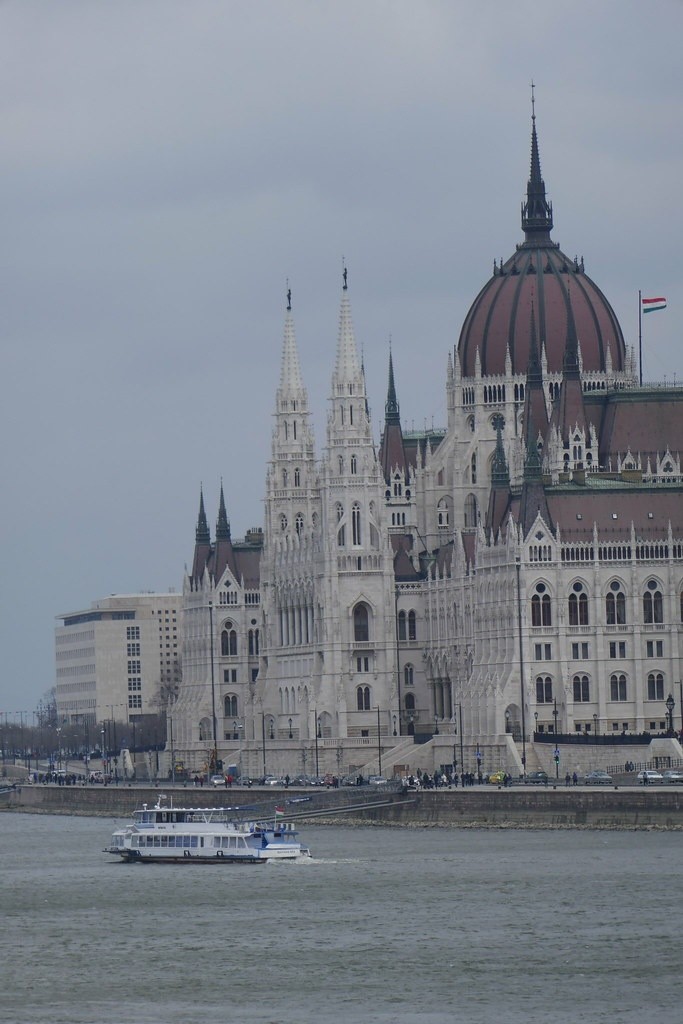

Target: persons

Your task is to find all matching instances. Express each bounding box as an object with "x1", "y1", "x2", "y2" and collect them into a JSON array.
[
  {"x1": 423, "y1": 771, "x2": 490, "y2": 789},
  {"x1": 194, "y1": 775, "x2": 199, "y2": 787},
  {"x1": 359, "y1": 774, "x2": 363, "y2": 784},
  {"x1": 200, "y1": 775, "x2": 204, "y2": 786},
  {"x1": 643, "y1": 772, "x2": 648, "y2": 786},
  {"x1": 417, "y1": 768, "x2": 422, "y2": 779},
  {"x1": 33, "y1": 772, "x2": 95, "y2": 786},
  {"x1": 224, "y1": 775, "x2": 232, "y2": 788},
  {"x1": 543, "y1": 774, "x2": 548, "y2": 787},
  {"x1": 625, "y1": 761, "x2": 634, "y2": 772},
  {"x1": 409, "y1": 775, "x2": 414, "y2": 787},
  {"x1": 573, "y1": 771, "x2": 577, "y2": 785},
  {"x1": 332, "y1": 776, "x2": 338, "y2": 788},
  {"x1": 168, "y1": 768, "x2": 172, "y2": 779},
  {"x1": 104, "y1": 775, "x2": 107, "y2": 787},
  {"x1": 503, "y1": 773, "x2": 512, "y2": 787},
  {"x1": 114, "y1": 775, "x2": 119, "y2": 787},
  {"x1": 286, "y1": 774, "x2": 289, "y2": 785},
  {"x1": 565, "y1": 772, "x2": 571, "y2": 787}
]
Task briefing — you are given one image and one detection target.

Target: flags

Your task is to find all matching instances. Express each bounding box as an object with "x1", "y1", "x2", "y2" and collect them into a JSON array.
[
  {"x1": 642, "y1": 297, "x2": 667, "y2": 314},
  {"x1": 276, "y1": 808, "x2": 284, "y2": 816}
]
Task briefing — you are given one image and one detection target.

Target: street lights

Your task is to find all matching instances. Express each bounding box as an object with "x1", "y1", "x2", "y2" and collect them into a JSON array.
[
  {"x1": 233, "y1": 713, "x2": 440, "y2": 739},
  {"x1": 132, "y1": 705, "x2": 382, "y2": 777},
  {"x1": 506, "y1": 697, "x2": 597, "y2": 736},
  {"x1": 666, "y1": 692, "x2": 675, "y2": 732},
  {"x1": 453, "y1": 702, "x2": 464, "y2": 775}
]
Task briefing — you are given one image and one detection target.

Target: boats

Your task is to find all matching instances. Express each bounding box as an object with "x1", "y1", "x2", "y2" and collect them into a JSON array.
[{"x1": 101, "y1": 793, "x2": 312, "y2": 863}]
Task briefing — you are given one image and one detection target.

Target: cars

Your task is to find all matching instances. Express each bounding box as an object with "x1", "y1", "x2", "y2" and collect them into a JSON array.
[
  {"x1": 584, "y1": 771, "x2": 613, "y2": 785},
  {"x1": 210, "y1": 775, "x2": 448, "y2": 787},
  {"x1": 637, "y1": 770, "x2": 683, "y2": 783},
  {"x1": 524, "y1": 771, "x2": 548, "y2": 784},
  {"x1": 52, "y1": 770, "x2": 103, "y2": 784}
]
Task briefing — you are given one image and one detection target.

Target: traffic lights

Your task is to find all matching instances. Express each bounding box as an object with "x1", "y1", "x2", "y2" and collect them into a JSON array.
[{"x1": 556, "y1": 757, "x2": 559, "y2": 765}]
